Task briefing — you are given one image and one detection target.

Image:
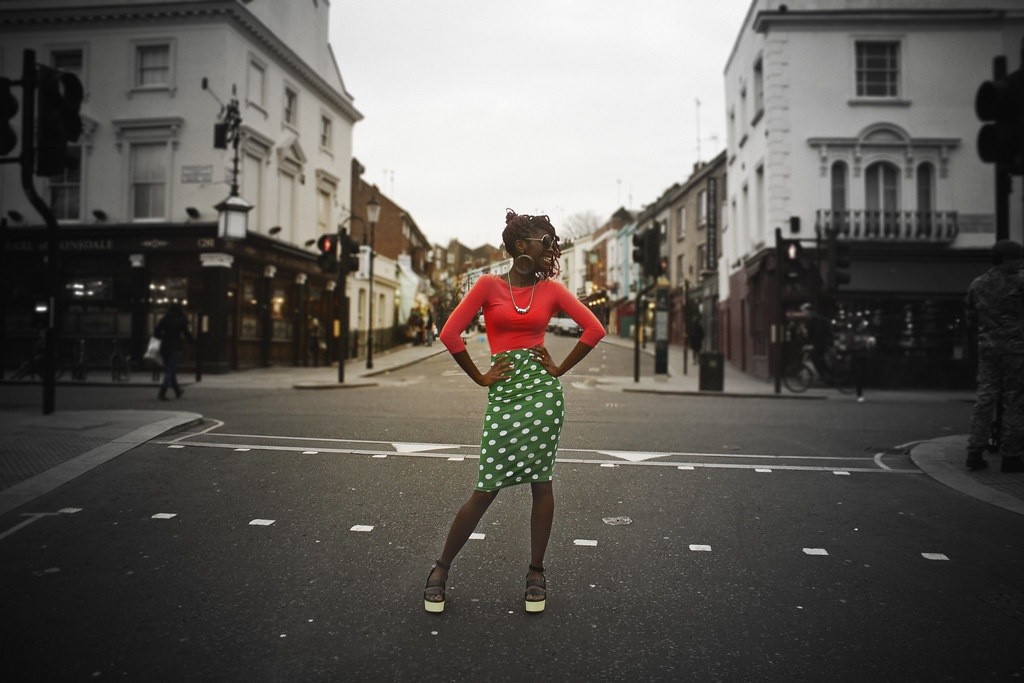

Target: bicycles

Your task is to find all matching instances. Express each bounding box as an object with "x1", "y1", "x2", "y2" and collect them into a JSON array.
[{"x1": 782, "y1": 343, "x2": 865, "y2": 396}]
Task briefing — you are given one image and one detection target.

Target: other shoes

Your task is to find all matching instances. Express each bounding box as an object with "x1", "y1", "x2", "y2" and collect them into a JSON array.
[
  {"x1": 966, "y1": 453, "x2": 988, "y2": 468},
  {"x1": 1002, "y1": 456, "x2": 1024, "y2": 472},
  {"x1": 176, "y1": 391, "x2": 184, "y2": 398},
  {"x1": 158, "y1": 397, "x2": 168, "y2": 401}
]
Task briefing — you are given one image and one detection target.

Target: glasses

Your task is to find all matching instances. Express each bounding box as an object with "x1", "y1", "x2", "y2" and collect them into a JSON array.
[{"x1": 523, "y1": 233, "x2": 557, "y2": 251}]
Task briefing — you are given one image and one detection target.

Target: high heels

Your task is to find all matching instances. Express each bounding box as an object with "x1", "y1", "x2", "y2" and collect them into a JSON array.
[
  {"x1": 525, "y1": 564, "x2": 546, "y2": 612},
  {"x1": 424, "y1": 559, "x2": 450, "y2": 612}
]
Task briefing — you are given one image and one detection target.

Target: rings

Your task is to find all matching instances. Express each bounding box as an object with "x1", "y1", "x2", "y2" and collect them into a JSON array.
[{"x1": 542, "y1": 355, "x2": 544, "y2": 357}]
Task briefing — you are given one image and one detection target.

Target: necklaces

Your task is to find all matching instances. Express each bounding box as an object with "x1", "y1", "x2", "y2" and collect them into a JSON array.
[{"x1": 507, "y1": 271, "x2": 536, "y2": 314}]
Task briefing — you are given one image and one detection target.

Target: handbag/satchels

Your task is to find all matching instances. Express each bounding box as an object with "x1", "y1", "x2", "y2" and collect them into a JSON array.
[{"x1": 143, "y1": 336, "x2": 164, "y2": 365}]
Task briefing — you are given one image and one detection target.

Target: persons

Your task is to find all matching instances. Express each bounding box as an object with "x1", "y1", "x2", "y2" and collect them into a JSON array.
[
  {"x1": 966, "y1": 240, "x2": 1024, "y2": 470},
  {"x1": 688, "y1": 316, "x2": 706, "y2": 363},
  {"x1": 424, "y1": 212, "x2": 605, "y2": 611},
  {"x1": 787, "y1": 303, "x2": 834, "y2": 380},
  {"x1": 406, "y1": 307, "x2": 433, "y2": 348},
  {"x1": 155, "y1": 303, "x2": 198, "y2": 400}
]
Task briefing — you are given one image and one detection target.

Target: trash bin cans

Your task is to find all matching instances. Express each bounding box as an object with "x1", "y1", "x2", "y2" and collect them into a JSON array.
[{"x1": 698, "y1": 350, "x2": 723, "y2": 390}]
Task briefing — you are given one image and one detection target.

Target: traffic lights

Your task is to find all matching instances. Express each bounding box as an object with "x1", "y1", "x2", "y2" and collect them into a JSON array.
[
  {"x1": 655, "y1": 220, "x2": 671, "y2": 276},
  {"x1": 974, "y1": 67, "x2": 1024, "y2": 176},
  {"x1": 317, "y1": 234, "x2": 340, "y2": 273},
  {"x1": 35, "y1": 70, "x2": 85, "y2": 178},
  {"x1": 1, "y1": 76, "x2": 18, "y2": 158},
  {"x1": 633, "y1": 228, "x2": 656, "y2": 274}
]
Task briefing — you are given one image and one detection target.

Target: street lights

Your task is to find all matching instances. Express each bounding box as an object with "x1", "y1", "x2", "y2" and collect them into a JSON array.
[{"x1": 364, "y1": 196, "x2": 382, "y2": 370}]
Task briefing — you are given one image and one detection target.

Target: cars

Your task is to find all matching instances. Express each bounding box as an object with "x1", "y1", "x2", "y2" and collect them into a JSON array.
[{"x1": 546, "y1": 317, "x2": 584, "y2": 338}]
